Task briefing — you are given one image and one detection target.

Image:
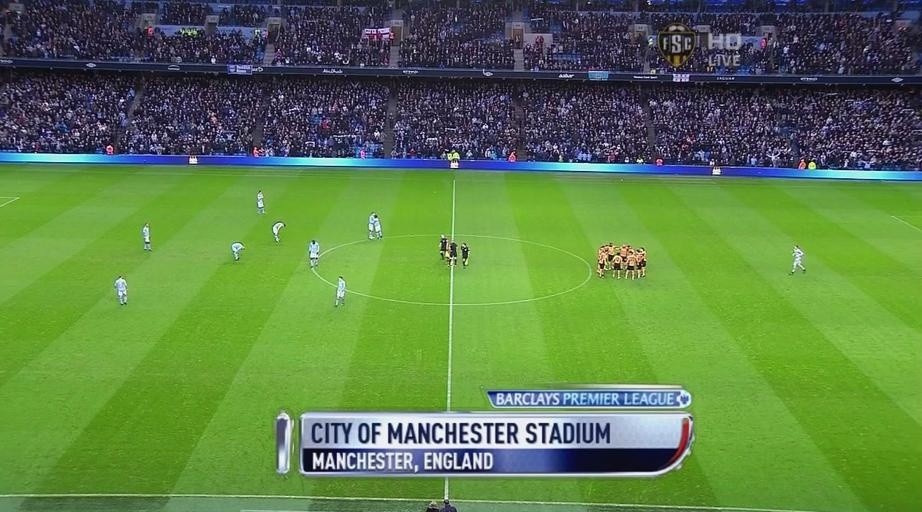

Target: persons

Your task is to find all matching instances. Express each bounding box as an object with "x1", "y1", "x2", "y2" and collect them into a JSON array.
[
  {"x1": 232, "y1": 242, "x2": 246, "y2": 262},
  {"x1": 425, "y1": 501, "x2": 440, "y2": 512},
  {"x1": 438, "y1": 234, "x2": 470, "y2": 267},
  {"x1": 596, "y1": 242, "x2": 647, "y2": 281},
  {"x1": 114, "y1": 276, "x2": 128, "y2": 305},
  {"x1": 368, "y1": 212, "x2": 376, "y2": 240},
  {"x1": 143, "y1": 223, "x2": 153, "y2": 251},
  {"x1": 308, "y1": 239, "x2": 320, "y2": 270},
  {"x1": 439, "y1": 499, "x2": 457, "y2": 512},
  {"x1": 372, "y1": 215, "x2": 384, "y2": 238},
  {"x1": 789, "y1": 245, "x2": 806, "y2": 274},
  {"x1": 272, "y1": 222, "x2": 286, "y2": 242},
  {"x1": 335, "y1": 276, "x2": 345, "y2": 307},
  {"x1": 256, "y1": 191, "x2": 266, "y2": 215}
]
